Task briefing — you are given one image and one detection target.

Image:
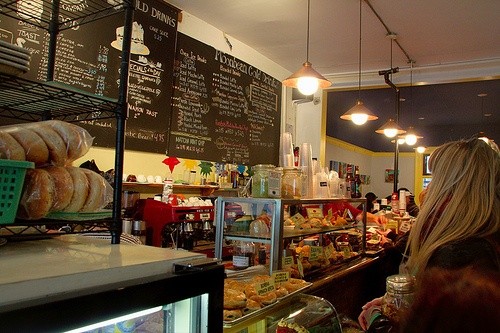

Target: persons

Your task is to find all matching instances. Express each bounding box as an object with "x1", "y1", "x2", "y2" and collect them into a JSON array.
[
  {"x1": 356, "y1": 191, "x2": 380, "y2": 214},
  {"x1": 405, "y1": 191, "x2": 419, "y2": 217},
  {"x1": 359, "y1": 139, "x2": 500, "y2": 333}
]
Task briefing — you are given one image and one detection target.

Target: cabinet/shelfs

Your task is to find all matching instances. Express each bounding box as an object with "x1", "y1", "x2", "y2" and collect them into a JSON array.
[{"x1": 213, "y1": 195, "x2": 368, "y2": 284}]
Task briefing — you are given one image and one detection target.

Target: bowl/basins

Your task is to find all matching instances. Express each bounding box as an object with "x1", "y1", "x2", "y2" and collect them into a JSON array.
[
  {"x1": 249, "y1": 219, "x2": 269, "y2": 237},
  {"x1": 123, "y1": 173, "x2": 162, "y2": 184}
]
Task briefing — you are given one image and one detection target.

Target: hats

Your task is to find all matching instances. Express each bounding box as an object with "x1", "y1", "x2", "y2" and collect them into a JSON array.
[{"x1": 366, "y1": 192, "x2": 377, "y2": 202}]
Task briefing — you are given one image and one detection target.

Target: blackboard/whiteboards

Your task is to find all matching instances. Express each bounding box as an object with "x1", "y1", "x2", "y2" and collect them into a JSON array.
[
  {"x1": 0, "y1": 0, "x2": 182, "y2": 156},
  {"x1": 170, "y1": 31, "x2": 282, "y2": 167}
]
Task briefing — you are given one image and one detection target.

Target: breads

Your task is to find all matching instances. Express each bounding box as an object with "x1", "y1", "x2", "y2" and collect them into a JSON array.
[
  {"x1": 247, "y1": 215, "x2": 393, "y2": 277},
  {"x1": 0, "y1": 120, "x2": 104, "y2": 218},
  {"x1": 224, "y1": 274, "x2": 307, "y2": 320}
]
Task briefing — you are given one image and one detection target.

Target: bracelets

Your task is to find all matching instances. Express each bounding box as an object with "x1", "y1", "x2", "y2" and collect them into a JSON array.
[{"x1": 369, "y1": 308, "x2": 381, "y2": 316}]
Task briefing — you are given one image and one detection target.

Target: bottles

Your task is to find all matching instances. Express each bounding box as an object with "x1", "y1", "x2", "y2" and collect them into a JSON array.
[
  {"x1": 381, "y1": 274, "x2": 417, "y2": 327},
  {"x1": 250, "y1": 164, "x2": 303, "y2": 201},
  {"x1": 314, "y1": 160, "x2": 347, "y2": 200},
  {"x1": 188, "y1": 171, "x2": 196, "y2": 185},
  {"x1": 162, "y1": 180, "x2": 174, "y2": 203}
]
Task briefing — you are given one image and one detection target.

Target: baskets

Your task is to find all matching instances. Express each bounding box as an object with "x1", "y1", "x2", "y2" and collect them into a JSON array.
[{"x1": 0, "y1": 159, "x2": 35, "y2": 225}]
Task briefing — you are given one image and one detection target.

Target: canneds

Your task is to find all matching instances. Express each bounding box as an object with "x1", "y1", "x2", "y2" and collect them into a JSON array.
[{"x1": 251, "y1": 164, "x2": 303, "y2": 200}]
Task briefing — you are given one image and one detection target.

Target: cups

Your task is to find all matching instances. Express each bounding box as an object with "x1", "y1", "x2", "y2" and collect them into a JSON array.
[
  {"x1": 398, "y1": 189, "x2": 406, "y2": 214},
  {"x1": 391, "y1": 193, "x2": 398, "y2": 214},
  {"x1": 279, "y1": 132, "x2": 295, "y2": 169},
  {"x1": 168, "y1": 195, "x2": 178, "y2": 207},
  {"x1": 299, "y1": 142, "x2": 313, "y2": 200}
]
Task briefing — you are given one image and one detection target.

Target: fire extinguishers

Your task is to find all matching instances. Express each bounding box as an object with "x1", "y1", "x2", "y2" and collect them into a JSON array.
[{"x1": 294, "y1": 147, "x2": 299, "y2": 166}]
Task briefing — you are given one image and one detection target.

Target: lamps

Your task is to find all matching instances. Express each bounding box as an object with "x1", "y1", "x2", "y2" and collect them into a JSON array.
[
  {"x1": 282, "y1": 1, "x2": 332, "y2": 96},
  {"x1": 340, "y1": 0, "x2": 432, "y2": 152}
]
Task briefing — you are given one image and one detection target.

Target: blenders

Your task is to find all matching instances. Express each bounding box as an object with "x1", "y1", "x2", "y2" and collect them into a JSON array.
[{"x1": 122, "y1": 190, "x2": 140, "y2": 221}]
